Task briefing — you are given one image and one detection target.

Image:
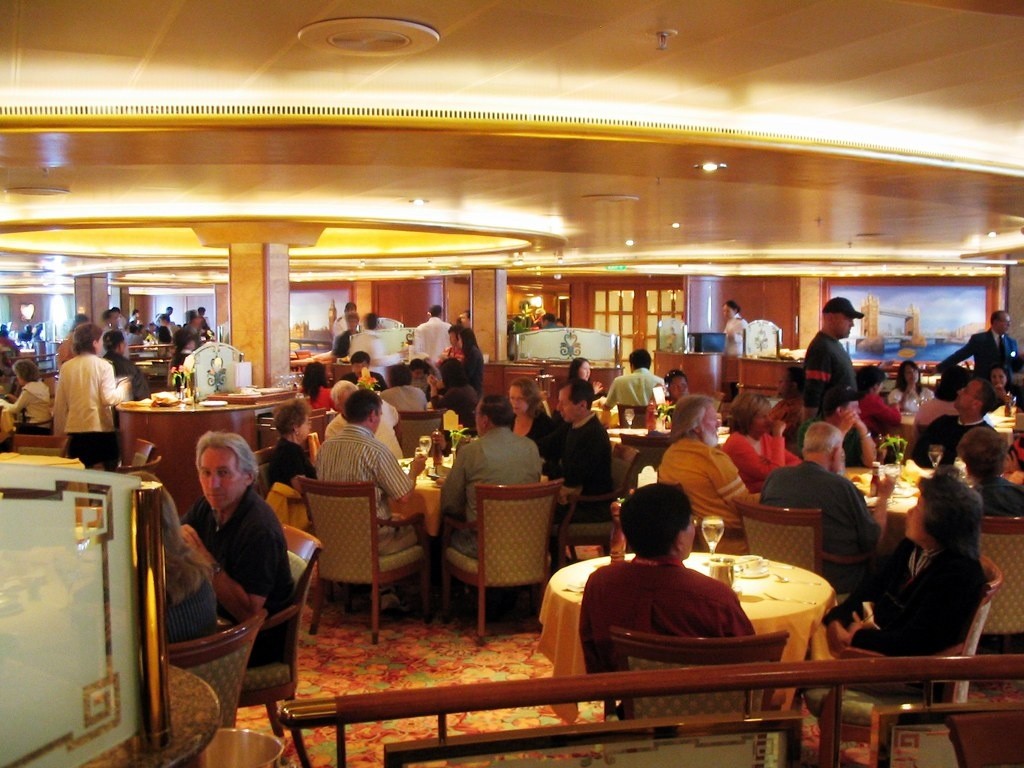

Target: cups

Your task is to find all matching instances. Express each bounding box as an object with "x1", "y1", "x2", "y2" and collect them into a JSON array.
[
  {"x1": 716, "y1": 413, "x2": 721, "y2": 429},
  {"x1": 76, "y1": 523, "x2": 91, "y2": 551},
  {"x1": 928, "y1": 445, "x2": 943, "y2": 468},
  {"x1": 709, "y1": 556, "x2": 733, "y2": 588},
  {"x1": 273, "y1": 372, "x2": 304, "y2": 390},
  {"x1": 953, "y1": 456, "x2": 969, "y2": 479},
  {"x1": 914, "y1": 396, "x2": 927, "y2": 408},
  {"x1": 740, "y1": 555, "x2": 768, "y2": 571}
]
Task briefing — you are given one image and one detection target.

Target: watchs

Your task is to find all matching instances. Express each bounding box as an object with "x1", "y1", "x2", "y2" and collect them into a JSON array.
[{"x1": 210, "y1": 563, "x2": 221, "y2": 574}]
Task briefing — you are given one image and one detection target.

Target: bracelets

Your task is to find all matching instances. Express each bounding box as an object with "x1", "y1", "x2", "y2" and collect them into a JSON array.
[{"x1": 861, "y1": 431, "x2": 871, "y2": 440}]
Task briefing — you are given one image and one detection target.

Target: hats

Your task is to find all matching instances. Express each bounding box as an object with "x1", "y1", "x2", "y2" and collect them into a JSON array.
[{"x1": 822, "y1": 297, "x2": 865, "y2": 319}]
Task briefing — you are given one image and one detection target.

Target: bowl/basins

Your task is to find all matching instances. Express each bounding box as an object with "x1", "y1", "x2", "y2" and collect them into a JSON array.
[
  {"x1": 247, "y1": 385, "x2": 259, "y2": 388},
  {"x1": 241, "y1": 388, "x2": 254, "y2": 394},
  {"x1": 918, "y1": 364, "x2": 926, "y2": 369}
]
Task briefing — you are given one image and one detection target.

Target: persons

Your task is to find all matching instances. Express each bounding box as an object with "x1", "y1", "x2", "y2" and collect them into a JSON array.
[
  {"x1": 578, "y1": 483, "x2": 756, "y2": 677},
  {"x1": 957, "y1": 426, "x2": 1024, "y2": 517},
  {"x1": 263, "y1": 349, "x2": 687, "y2": 615},
  {"x1": 0, "y1": 358, "x2": 52, "y2": 435},
  {"x1": 170, "y1": 329, "x2": 200, "y2": 392},
  {"x1": 130, "y1": 430, "x2": 294, "y2": 667},
  {"x1": 722, "y1": 300, "x2": 748, "y2": 397},
  {"x1": 100, "y1": 305, "x2": 214, "y2": 359},
  {"x1": 823, "y1": 476, "x2": 988, "y2": 698},
  {"x1": 541, "y1": 313, "x2": 555, "y2": 328},
  {"x1": 53, "y1": 323, "x2": 132, "y2": 472},
  {"x1": 331, "y1": 302, "x2": 485, "y2": 398},
  {"x1": 802, "y1": 296, "x2": 876, "y2": 468},
  {"x1": 656, "y1": 365, "x2": 803, "y2": 555},
  {"x1": 101, "y1": 329, "x2": 152, "y2": 429},
  {"x1": 0, "y1": 324, "x2": 33, "y2": 353},
  {"x1": 856, "y1": 310, "x2": 1024, "y2": 469},
  {"x1": 56, "y1": 314, "x2": 88, "y2": 369}
]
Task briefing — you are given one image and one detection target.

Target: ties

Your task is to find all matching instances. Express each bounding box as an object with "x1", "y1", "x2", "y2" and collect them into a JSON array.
[{"x1": 999, "y1": 336, "x2": 1005, "y2": 362}]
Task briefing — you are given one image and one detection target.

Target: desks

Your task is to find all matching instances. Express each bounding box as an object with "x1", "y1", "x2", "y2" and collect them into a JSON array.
[
  {"x1": 539, "y1": 552, "x2": 839, "y2": 725},
  {"x1": 0, "y1": 453, "x2": 88, "y2": 505},
  {"x1": 607, "y1": 428, "x2": 730, "y2": 443},
  {"x1": 19, "y1": 348, "x2": 35, "y2": 357},
  {"x1": 839, "y1": 466, "x2": 935, "y2": 549},
  {"x1": 592, "y1": 400, "x2": 622, "y2": 428},
  {"x1": 893, "y1": 412, "x2": 1016, "y2": 450},
  {"x1": 386, "y1": 457, "x2": 455, "y2": 536}
]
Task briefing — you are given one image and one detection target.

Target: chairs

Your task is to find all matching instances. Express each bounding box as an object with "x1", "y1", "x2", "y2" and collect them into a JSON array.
[
  {"x1": 236, "y1": 523, "x2": 324, "y2": 768},
  {"x1": 251, "y1": 408, "x2": 1024, "y2": 768},
  {"x1": 167, "y1": 605, "x2": 266, "y2": 728},
  {"x1": 0, "y1": 334, "x2": 171, "y2": 504}
]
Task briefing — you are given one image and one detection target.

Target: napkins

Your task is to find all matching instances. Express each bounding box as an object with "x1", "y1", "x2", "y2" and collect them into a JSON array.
[
  {"x1": 46, "y1": 458, "x2": 79, "y2": 464},
  {"x1": 0, "y1": 453, "x2": 19, "y2": 460}
]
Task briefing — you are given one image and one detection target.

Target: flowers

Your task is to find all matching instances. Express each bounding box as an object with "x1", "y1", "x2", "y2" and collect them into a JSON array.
[
  {"x1": 881, "y1": 433, "x2": 908, "y2": 455},
  {"x1": 510, "y1": 303, "x2": 546, "y2": 332},
  {"x1": 448, "y1": 423, "x2": 470, "y2": 448},
  {"x1": 357, "y1": 376, "x2": 382, "y2": 390},
  {"x1": 170, "y1": 365, "x2": 196, "y2": 387},
  {"x1": 655, "y1": 401, "x2": 675, "y2": 420}
]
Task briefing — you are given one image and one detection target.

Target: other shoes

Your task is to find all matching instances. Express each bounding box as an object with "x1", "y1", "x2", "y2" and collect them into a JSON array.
[{"x1": 379, "y1": 592, "x2": 401, "y2": 611}]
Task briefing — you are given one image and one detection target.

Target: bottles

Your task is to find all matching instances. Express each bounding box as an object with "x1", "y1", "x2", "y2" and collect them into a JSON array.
[
  {"x1": 870, "y1": 462, "x2": 880, "y2": 497},
  {"x1": 1005, "y1": 391, "x2": 1011, "y2": 417},
  {"x1": 179, "y1": 386, "x2": 185, "y2": 408}
]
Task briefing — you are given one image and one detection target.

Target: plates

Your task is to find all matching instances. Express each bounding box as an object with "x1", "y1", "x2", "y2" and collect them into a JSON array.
[
  {"x1": 730, "y1": 565, "x2": 771, "y2": 578},
  {"x1": 864, "y1": 497, "x2": 895, "y2": 507},
  {"x1": 200, "y1": 401, "x2": 228, "y2": 406},
  {"x1": 227, "y1": 393, "x2": 260, "y2": 396},
  {"x1": 256, "y1": 388, "x2": 284, "y2": 393}
]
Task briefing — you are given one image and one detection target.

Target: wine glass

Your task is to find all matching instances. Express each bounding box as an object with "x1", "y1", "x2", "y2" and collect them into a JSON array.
[
  {"x1": 884, "y1": 464, "x2": 901, "y2": 506},
  {"x1": 414, "y1": 436, "x2": 431, "y2": 479},
  {"x1": 701, "y1": 516, "x2": 724, "y2": 561},
  {"x1": 624, "y1": 408, "x2": 635, "y2": 432}
]
